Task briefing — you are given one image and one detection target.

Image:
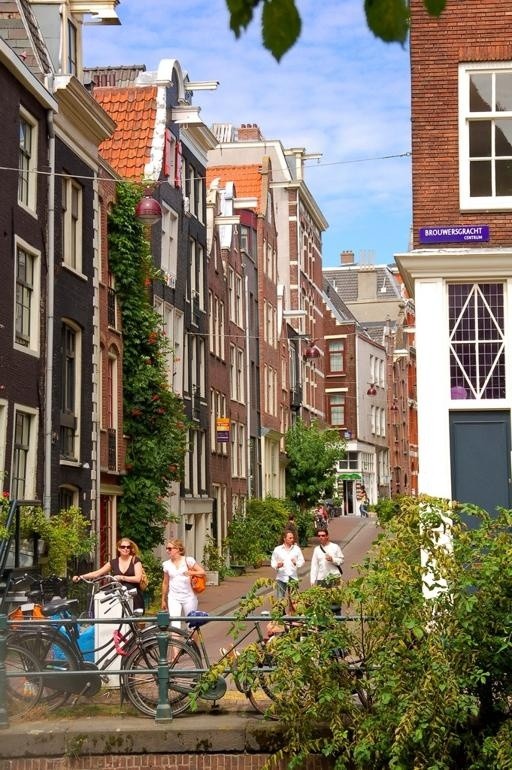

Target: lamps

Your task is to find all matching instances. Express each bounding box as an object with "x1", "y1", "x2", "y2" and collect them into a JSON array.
[
  {"x1": 381, "y1": 277, "x2": 386, "y2": 292},
  {"x1": 367, "y1": 382, "x2": 378, "y2": 396},
  {"x1": 333, "y1": 280, "x2": 337, "y2": 292},
  {"x1": 305, "y1": 337, "x2": 320, "y2": 359},
  {"x1": 135, "y1": 181, "x2": 162, "y2": 226}
]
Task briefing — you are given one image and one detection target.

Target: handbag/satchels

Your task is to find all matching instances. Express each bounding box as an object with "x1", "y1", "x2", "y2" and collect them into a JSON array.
[
  {"x1": 132, "y1": 555, "x2": 149, "y2": 591},
  {"x1": 185, "y1": 556, "x2": 206, "y2": 593}
]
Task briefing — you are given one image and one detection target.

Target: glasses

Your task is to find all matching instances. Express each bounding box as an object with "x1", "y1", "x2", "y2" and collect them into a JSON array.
[
  {"x1": 166, "y1": 546, "x2": 179, "y2": 551},
  {"x1": 317, "y1": 534, "x2": 326, "y2": 537},
  {"x1": 119, "y1": 546, "x2": 131, "y2": 549}
]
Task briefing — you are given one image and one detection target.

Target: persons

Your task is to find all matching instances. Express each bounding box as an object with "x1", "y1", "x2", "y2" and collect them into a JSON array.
[
  {"x1": 71, "y1": 537, "x2": 147, "y2": 617},
  {"x1": 358, "y1": 490, "x2": 369, "y2": 519},
  {"x1": 312, "y1": 500, "x2": 330, "y2": 532},
  {"x1": 360, "y1": 485, "x2": 367, "y2": 518},
  {"x1": 161, "y1": 538, "x2": 208, "y2": 662},
  {"x1": 285, "y1": 514, "x2": 301, "y2": 547},
  {"x1": 270, "y1": 529, "x2": 305, "y2": 616},
  {"x1": 310, "y1": 527, "x2": 345, "y2": 615}
]
